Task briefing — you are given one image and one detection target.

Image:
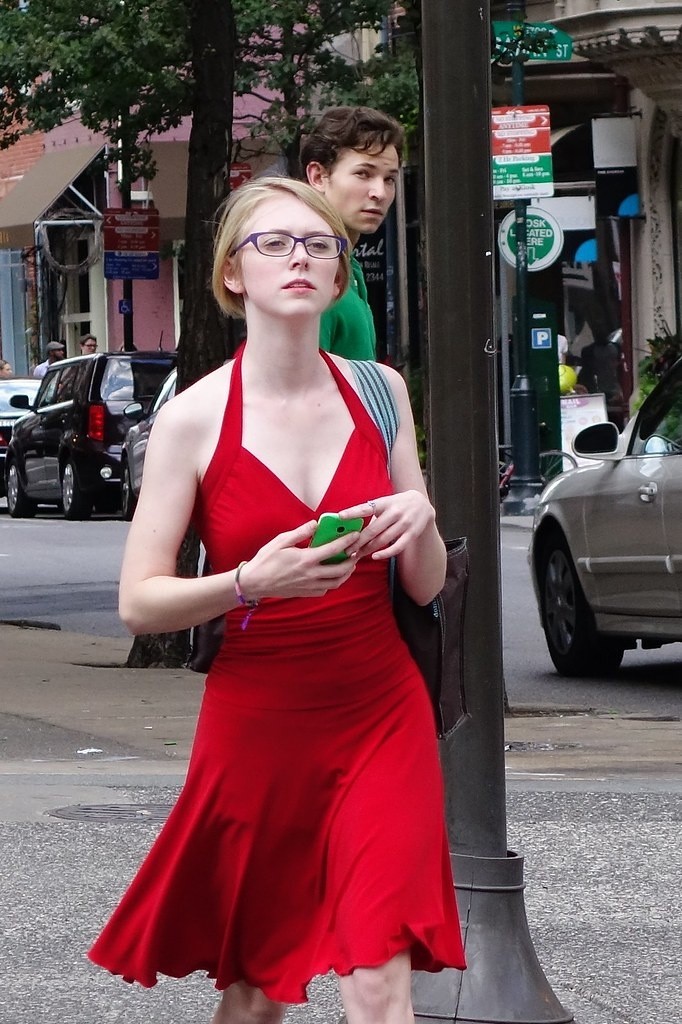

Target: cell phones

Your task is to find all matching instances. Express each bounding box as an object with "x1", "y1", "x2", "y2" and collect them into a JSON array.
[{"x1": 306, "y1": 513, "x2": 364, "y2": 566}]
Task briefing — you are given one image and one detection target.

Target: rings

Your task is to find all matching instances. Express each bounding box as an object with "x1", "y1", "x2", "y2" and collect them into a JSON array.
[{"x1": 367, "y1": 501, "x2": 377, "y2": 514}]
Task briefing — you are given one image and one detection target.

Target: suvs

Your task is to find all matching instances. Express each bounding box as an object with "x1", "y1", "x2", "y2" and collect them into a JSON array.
[{"x1": 3, "y1": 352, "x2": 176, "y2": 519}]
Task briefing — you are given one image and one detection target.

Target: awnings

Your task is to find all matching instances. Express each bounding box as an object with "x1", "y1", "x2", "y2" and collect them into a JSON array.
[
  {"x1": 0, "y1": 143, "x2": 106, "y2": 247},
  {"x1": 141, "y1": 140, "x2": 279, "y2": 239}
]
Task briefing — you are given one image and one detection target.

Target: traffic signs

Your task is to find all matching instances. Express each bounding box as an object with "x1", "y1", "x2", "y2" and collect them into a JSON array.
[{"x1": 490, "y1": 20, "x2": 575, "y2": 61}]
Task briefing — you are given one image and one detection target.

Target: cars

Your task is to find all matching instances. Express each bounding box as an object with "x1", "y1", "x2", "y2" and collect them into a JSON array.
[
  {"x1": 121, "y1": 366, "x2": 180, "y2": 520},
  {"x1": 526, "y1": 356, "x2": 682, "y2": 679},
  {"x1": 0, "y1": 377, "x2": 57, "y2": 498}
]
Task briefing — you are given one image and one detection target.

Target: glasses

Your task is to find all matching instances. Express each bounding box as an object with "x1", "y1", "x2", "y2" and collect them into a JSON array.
[
  {"x1": 228, "y1": 231, "x2": 349, "y2": 259},
  {"x1": 84, "y1": 344, "x2": 98, "y2": 350}
]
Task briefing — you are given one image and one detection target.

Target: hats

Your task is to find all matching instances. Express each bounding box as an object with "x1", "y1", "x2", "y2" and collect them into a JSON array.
[{"x1": 46, "y1": 341, "x2": 64, "y2": 354}]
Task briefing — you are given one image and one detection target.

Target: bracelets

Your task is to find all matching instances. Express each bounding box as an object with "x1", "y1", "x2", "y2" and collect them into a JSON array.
[{"x1": 234, "y1": 561, "x2": 260, "y2": 630}]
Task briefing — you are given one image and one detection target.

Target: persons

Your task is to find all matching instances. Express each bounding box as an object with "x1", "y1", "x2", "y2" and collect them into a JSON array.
[
  {"x1": 0, "y1": 359, "x2": 12, "y2": 378},
  {"x1": 86, "y1": 177, "x2": 467, "y2": 1024},
  {"x1": 300, "y1": 106, "x2": 407, "y2": 362},
  {"x1": 579, "y1": 321, "x2": 622, "y2": 391},
  {"x1": 80, "y1": 333, "x2": 98, "y2": 354},
  {"x1": 33, "y1": 341, "x2": 65, "y2": 376},
  {"x1": 558, "y1": 334, "x2": 568, "y2": 363}
]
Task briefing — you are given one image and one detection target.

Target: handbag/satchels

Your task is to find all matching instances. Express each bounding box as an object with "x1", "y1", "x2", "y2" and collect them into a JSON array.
[{"x1": 349, "y1": 356, "x2": 447, "y2": 737}]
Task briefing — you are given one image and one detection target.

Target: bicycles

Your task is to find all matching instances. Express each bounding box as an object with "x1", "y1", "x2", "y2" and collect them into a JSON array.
[{"x1": 497, "y1": 442, "x2": 577, "y2": 510}]
